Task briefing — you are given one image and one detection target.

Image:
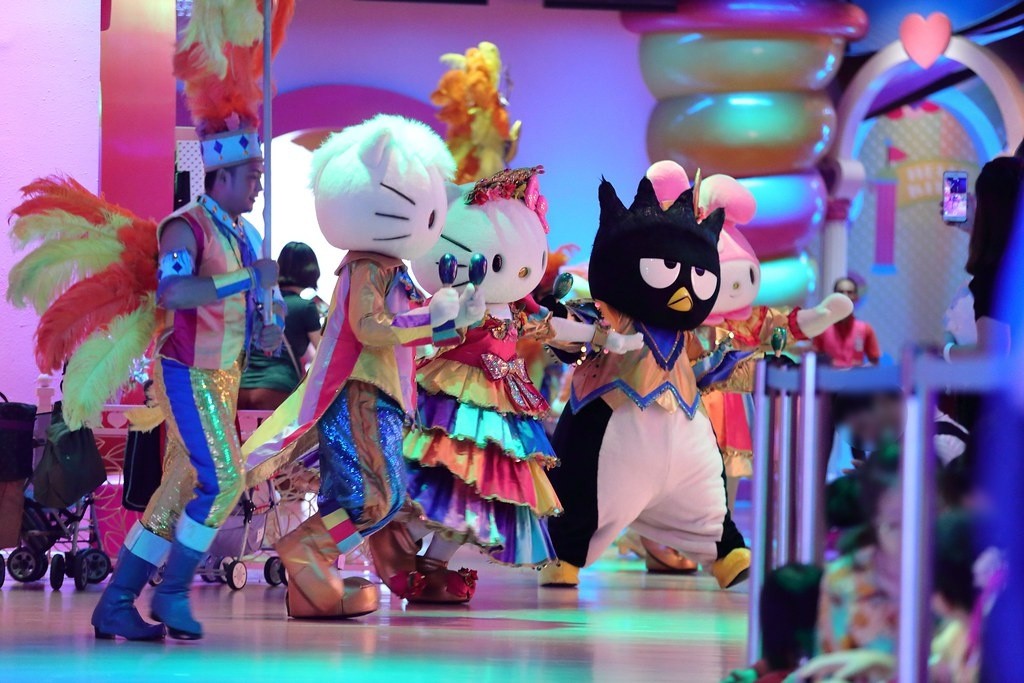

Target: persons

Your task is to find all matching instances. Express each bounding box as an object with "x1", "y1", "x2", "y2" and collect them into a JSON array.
[
  {"x1": 812, "y1": 276, "x2": 880, "y2": 485},
  {"x1": 91, "y1": 110, "x2": 280, "y2": 641},
  {"x1": 237, "y1": 241, "x2": 321, "y2": 411},
  {"x1": 819, "y1": 476, "x2": 985, "y2": 683},
  {"x1": 825, "y1": 341, "x2": 969, "y2": 486},
  {"x1": 935, "y1": 136, "x2": 1024, "y2": 365}
]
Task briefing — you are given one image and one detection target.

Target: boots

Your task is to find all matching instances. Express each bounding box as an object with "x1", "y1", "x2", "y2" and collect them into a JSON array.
[
  {"x1": 150, "y1": 509, "x2": 220, "y2": 639},
  {"x1": 91, "y1": 519, "x2": 166, "y2": 639}
]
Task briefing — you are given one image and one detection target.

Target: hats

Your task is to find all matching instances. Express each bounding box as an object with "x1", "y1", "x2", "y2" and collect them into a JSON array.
[{"x1": 172, "y1": 0, "x2": 294, "y2": 172}]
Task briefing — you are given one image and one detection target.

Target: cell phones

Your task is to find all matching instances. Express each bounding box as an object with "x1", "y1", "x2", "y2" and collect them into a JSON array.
[{"x1": 942, "y1": 171, "x2": 968, "y2": 223}]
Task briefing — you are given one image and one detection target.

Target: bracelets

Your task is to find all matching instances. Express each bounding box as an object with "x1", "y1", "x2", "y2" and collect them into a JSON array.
[
  {"x1": 210, "y1": 265, "x2": 258, "y2": 299},
  {"x1": 943, "y1": 343, "x2": 954, "y2": 364},
  {"x1": 943, "y1": 342, "x2": 954, "y2": 363}
]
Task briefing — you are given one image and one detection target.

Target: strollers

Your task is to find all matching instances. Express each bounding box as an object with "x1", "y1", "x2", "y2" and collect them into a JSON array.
[
  {"x1": 194, "y1": 478, "x2": 290, "y2": 593},
  {"x1": 5, "y1": 406, "x2": 111, "y2": 590}
]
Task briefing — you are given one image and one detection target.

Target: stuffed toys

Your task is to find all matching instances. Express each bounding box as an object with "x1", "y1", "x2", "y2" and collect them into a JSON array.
[{"x1": 272, "y1": 112, "x2": 854, "y2": 619}]
